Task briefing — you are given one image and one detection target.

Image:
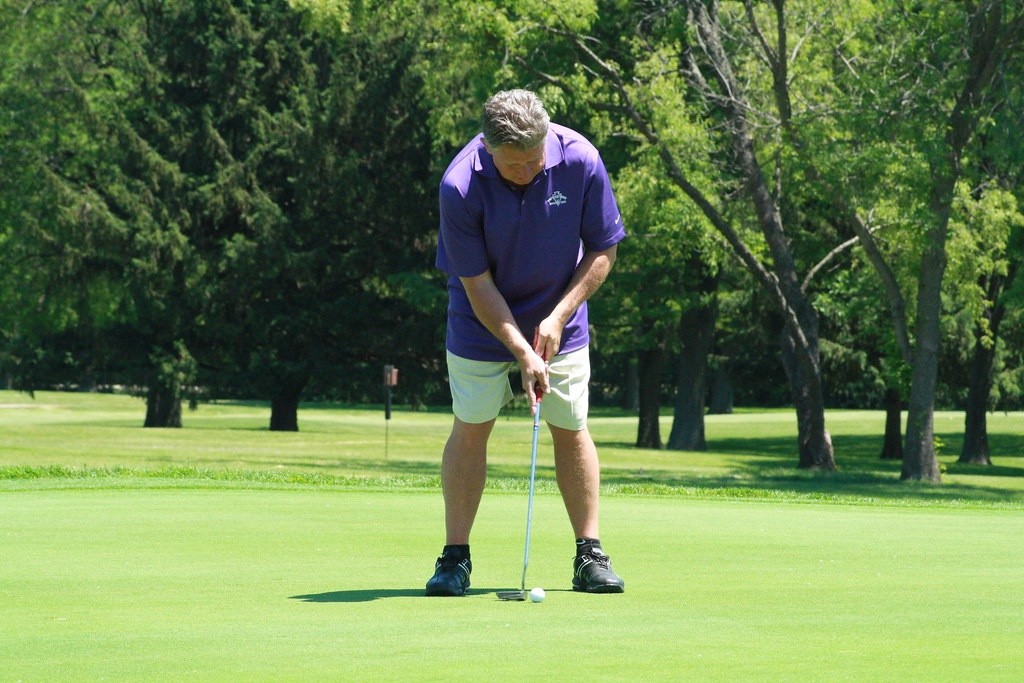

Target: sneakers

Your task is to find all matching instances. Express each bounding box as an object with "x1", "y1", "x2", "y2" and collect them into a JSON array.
[
  {"x1": 425, "y1": 548, "x2": 472, "y2": 596},
  {"x1": 572, "y1": 548, "x2": 624, "y2": 593}
]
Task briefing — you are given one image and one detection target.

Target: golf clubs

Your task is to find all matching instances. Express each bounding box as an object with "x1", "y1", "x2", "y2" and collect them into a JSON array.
[{"x1": 496, "y1": 341, "x2": 546, "y2": 599}]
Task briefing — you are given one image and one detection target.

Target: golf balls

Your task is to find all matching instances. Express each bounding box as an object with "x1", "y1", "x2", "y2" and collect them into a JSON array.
[{"x1": 530, "y1": 586, "x2": 545, "y2": 602}]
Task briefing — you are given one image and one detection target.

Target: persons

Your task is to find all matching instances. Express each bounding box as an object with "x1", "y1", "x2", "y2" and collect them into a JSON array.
[{"x1": 425, "y1": 89, "x2": 626, "y2": 597}]
